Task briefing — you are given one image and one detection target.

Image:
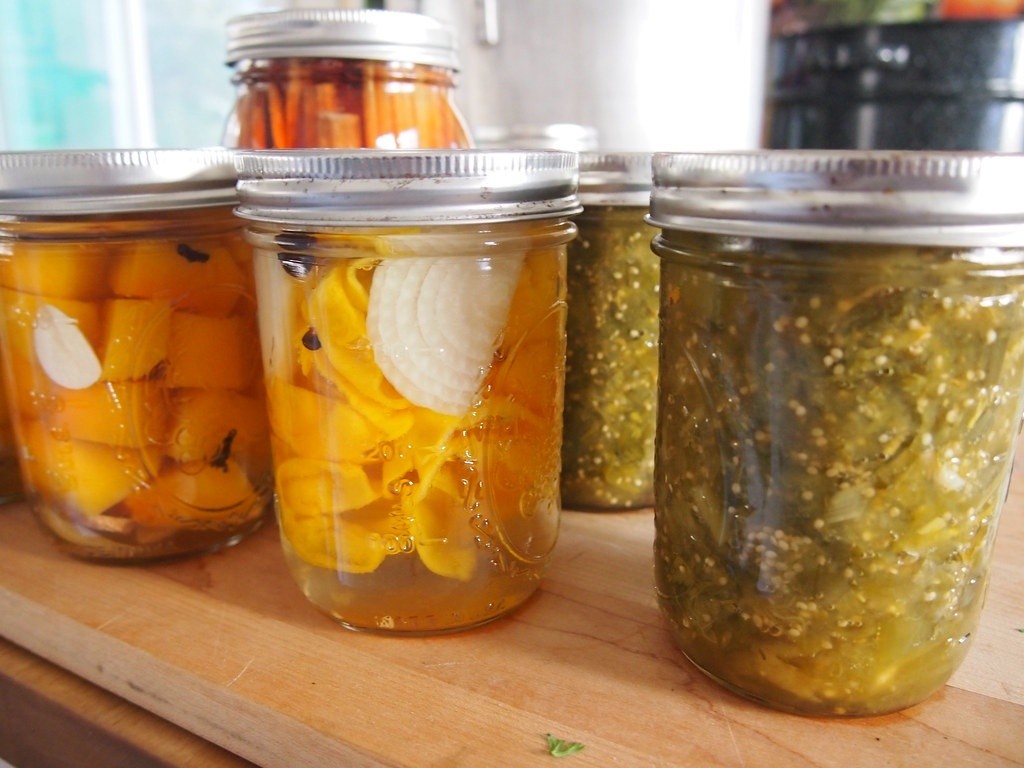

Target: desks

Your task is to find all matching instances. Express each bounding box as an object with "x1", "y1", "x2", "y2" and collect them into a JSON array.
[{"x1": 0, "y1": 407, "x2": 1024, "y2": 768}]
